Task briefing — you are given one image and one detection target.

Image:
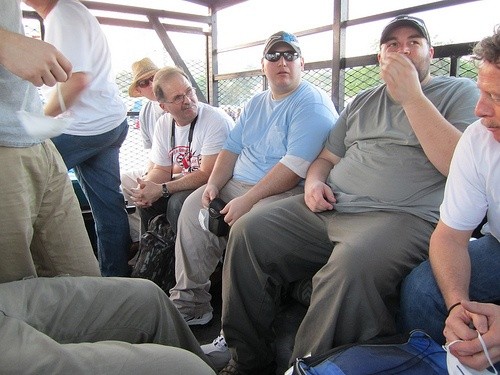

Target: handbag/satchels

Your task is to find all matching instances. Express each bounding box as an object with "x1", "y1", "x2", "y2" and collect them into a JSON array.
[
  {"x1": 291, "y1": 327, "x2": 447, "y2": 375},
  {"x1": 209, "y1": 198, "x2": 230, "y2": 237}
]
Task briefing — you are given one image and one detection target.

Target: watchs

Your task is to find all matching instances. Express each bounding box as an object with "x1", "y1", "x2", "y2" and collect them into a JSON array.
[{"x1": 162, "y1": 183, "x2": 168, "y2": 198}]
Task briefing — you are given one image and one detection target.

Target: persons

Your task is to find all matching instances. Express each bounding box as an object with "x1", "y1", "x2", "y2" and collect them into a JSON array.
[
  {"x1": 0, "y1": 278, "x2": 219, "y2": 375},
  {"x1": 200, "y1": 17, "x2": 482, "y2": 375},
  {"x1": 400, "y1": 30, "x2": 500, "y2": 375},
  {"x1": 119, "y1": 56, "x2": 161, "y2": 265},
  {"x1": 21, "y1": 0, "x2": 131, "y2": 277},
  {"x1": 0, "y1": 0, "x2": 101, "y2": 285},
  {"x1": 125, "y1": 65, "x2": 235, "y2": 237}
]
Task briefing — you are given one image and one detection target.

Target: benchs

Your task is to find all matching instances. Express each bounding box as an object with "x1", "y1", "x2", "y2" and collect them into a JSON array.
[{"x1": 78, "y1": 203, "x2": 136, "y2": 221}]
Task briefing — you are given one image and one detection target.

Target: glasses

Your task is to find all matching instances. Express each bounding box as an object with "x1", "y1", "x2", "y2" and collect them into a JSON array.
[
  {"x1": 165, "y1": 87, "x2": 197, "y2": 105},
  {"x1": 136, "y1": 75, "x2": 155, "y2": 88},
  {"x1": 265, "y1": 50, "x2": 300, "y2": 63}
]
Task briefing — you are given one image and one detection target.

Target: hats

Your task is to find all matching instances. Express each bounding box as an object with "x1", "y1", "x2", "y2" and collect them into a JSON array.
[
  {"x1": 380, "y1": 16, "x2": 431, "y2": 49},
  {"x1": 128, "y1": 57, "x2": 161, "y2": 98},
  {"x1": 263, "y1": 31, "x2": 301, "y2": 56}
]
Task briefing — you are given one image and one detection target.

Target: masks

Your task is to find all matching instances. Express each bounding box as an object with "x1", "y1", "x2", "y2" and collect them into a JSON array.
[
  {"x1": 198, "y1": 208, "x2": 210, "y2": 233},
  {"x1": 442, "y1": 331, "x2": 498, "y2": 375}
]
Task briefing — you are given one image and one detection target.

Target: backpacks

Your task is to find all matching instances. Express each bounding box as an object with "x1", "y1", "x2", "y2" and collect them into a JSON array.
[{"x1": 130, "y1": 213, "x2": 175, "y2": 298}]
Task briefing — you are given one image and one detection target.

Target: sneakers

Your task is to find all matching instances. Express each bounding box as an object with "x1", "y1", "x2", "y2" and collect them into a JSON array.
[
  {"x1": 185, "y1": 305, "x2": 213, "y2": 325},
  {"x1": 218, "y1": 359, "x2": 279, "y2": 375},
  {"x1": 200, "y1": 330, "x2": 229, "y2": 355}
]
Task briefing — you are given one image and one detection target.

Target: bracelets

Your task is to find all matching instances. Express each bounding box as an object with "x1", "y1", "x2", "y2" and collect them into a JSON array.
[{"x1": 448, "y1": 302, "x2": 460, "y2": 312}]
